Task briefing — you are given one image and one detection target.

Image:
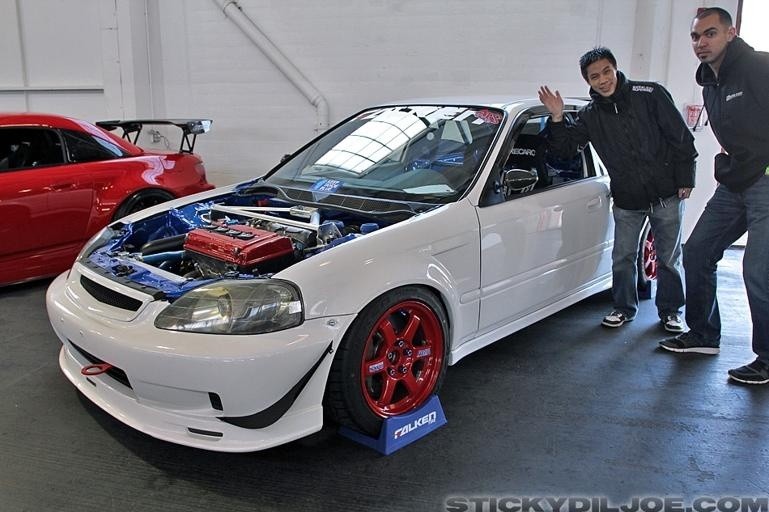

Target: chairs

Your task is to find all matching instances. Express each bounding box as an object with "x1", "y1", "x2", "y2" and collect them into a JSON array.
[{"x1": 502, "y1": 133, "x2": 549, "y2": 194}]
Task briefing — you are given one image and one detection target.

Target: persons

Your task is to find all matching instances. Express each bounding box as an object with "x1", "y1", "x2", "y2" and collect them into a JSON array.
[
  {"x1": 535, "y1": 46, "x2": 697, "y2": 332},
  {"x1": 658, "y1": 4, "x2": 768, "y2": 386}
]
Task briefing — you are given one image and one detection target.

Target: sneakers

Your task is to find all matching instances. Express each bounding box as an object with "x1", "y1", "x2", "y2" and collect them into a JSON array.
[
  {"x1": 656, "y1": 326, "x2": 722, "y2": 357},
  {"x1": 727, "y1": 357, "x2": 769, "y2": 386},
  {"x1": 600, "y1": 309, "x2": 636, "y2": 330},
  {"x1": 659, "y1": 313, "x2": 685, "y2": 333}
]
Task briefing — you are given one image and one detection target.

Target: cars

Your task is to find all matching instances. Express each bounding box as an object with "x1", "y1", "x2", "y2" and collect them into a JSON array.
[
  {"x1": 45, "y1": 97, "x2": 663, "y2": 456},
  {"x1": 0, "y1": 112, "x2": 216, "y2": 290}
]
